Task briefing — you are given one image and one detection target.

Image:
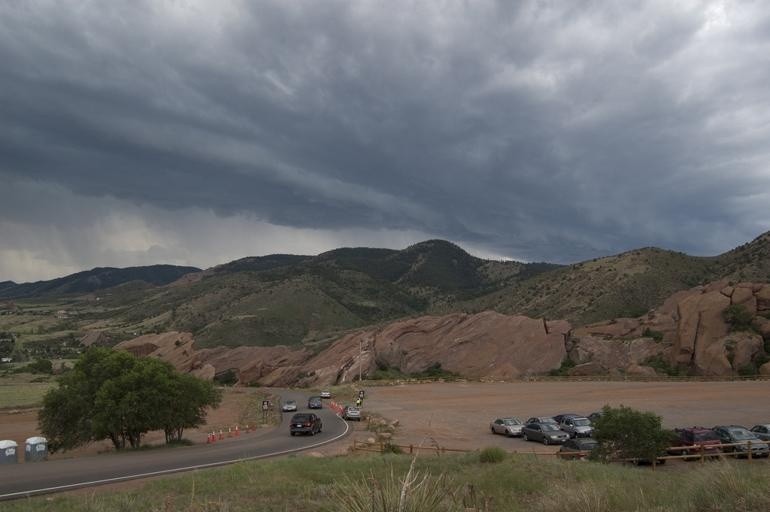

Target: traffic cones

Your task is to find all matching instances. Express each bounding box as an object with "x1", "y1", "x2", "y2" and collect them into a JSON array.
[
  {"x1": 228, "y1": 426, "x2": 234, "y2": 438},
  {"x1": 208, "y1": 432, "x2": 217, "y2": 443},
  {"x1": 235, "y1": 425, "x2": 241, "y2": 436},
  {"x1": 329, "y1": 400, "x2": 344, "y2": 415},
  {"x1": 219, "y1": 429, "x2": 224, "y2": 440}
]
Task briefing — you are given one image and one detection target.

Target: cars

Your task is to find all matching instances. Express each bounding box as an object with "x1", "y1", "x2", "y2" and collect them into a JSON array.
[
  {"x1": 342, "y1": 406, "x2": 360, "y2": 420},
  {"x1": 283, "y1": 399, "x2": 298, "y2": 411},
  {"x1": 490, "y1": 413, "x2": 606, "y2": 445},
  {"x1": 561, "y1": 438, "x2": 600, "y2": 460},
  {"x1": 290, "y1": 413, "x2": 323, "y2": 436},
  {"x1": 669, "y1": 424, "x2": 770, "y2": 461},
  {"x1": 321, "y1": 390, "x2": 331, "y2": 398},
  {"x1": 308, "y1": 396, "x2": 322, "y2": 409}
]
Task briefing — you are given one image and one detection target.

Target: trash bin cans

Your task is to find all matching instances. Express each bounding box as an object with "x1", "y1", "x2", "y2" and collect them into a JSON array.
[
  {"x1": 0, "y1": 439, "x2": 19, "y2": 466},
  {"x1": 25, "y1": 437, "x2": 48, "y2": 462}
]
